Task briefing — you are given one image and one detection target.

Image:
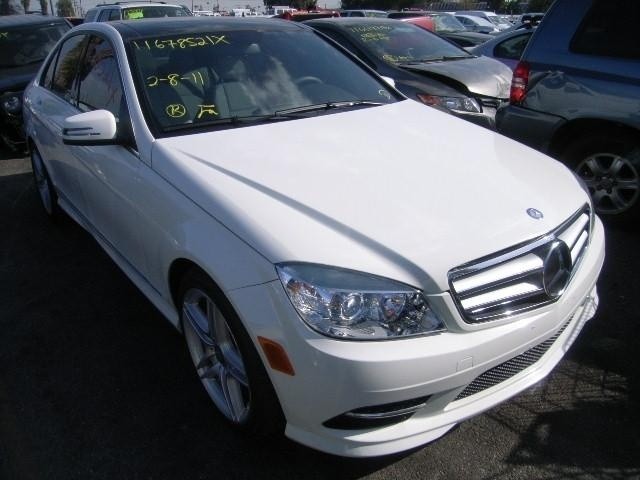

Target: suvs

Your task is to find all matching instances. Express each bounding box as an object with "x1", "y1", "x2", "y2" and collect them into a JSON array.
[{"x1": 493, "y1": 2, "x2": 640, "y2": 224}]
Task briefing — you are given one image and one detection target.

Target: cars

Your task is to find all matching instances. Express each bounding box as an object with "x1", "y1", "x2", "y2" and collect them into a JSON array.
[
  {"x1": 0, "y1": 13, "x2": 606, "y2": 460},
  {"x1": 0, "y1": 0, "x2": 541, "y2": 158}
]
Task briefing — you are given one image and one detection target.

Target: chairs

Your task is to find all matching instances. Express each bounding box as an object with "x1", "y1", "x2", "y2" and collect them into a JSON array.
[{"x1": 212, "y1": 59, "x2": 267, "y2": 117}]
